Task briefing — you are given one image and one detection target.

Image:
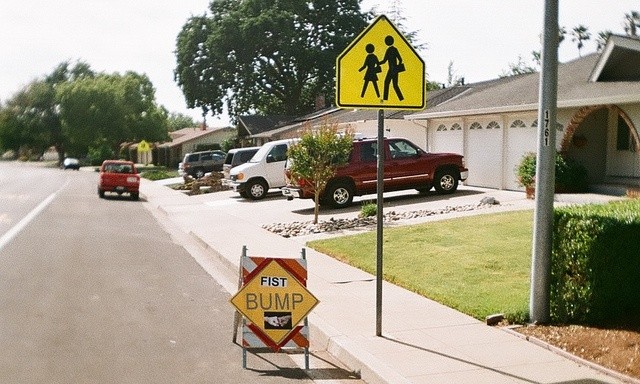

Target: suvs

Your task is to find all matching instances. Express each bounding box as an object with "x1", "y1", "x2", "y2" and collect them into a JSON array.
[
  {"x1": 220, "y1": 147, "x2": 260, "y2": 188},
  {"x1": 283, "y1": 139, "x2": 470, "y2": 208},
  {"x1": 178, "y1": 151, "x2": 229, "y2": 178}
]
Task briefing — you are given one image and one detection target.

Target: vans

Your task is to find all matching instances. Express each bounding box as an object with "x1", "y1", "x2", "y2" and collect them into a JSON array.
[{"x1": 226, "y1": 133, "x2": 365, "y2": 199}]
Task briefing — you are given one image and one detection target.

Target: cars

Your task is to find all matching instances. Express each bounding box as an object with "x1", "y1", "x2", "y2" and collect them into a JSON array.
[{"x1": 64, "y1": 158, "x2": 80, "y2": 170}]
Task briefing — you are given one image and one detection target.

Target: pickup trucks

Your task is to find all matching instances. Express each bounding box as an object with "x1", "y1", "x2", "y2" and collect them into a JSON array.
[{"x1": 96, "y1": 158, "x2": 140, "y2": 199}]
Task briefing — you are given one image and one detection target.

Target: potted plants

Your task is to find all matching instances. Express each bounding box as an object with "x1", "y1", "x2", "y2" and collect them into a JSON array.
[{"x1": 516, "y1": 154, "x2": 536, "y2": 199}]
